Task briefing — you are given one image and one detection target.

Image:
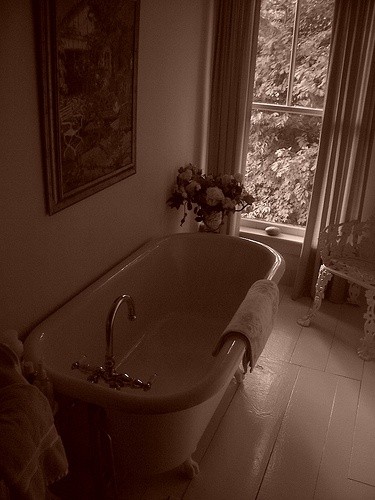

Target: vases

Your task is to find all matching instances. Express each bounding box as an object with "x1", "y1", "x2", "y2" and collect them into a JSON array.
[{"x1": 203, "y1": 209, "x2": 223, "y2": 231}]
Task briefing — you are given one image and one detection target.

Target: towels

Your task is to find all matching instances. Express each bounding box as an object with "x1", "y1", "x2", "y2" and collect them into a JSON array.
[
  {"x1": 211, "y1": 278, "x2": 285, "y2": 374},
  {"x1": 0, "y1": 380, "x2": 68, "y2": 500}
]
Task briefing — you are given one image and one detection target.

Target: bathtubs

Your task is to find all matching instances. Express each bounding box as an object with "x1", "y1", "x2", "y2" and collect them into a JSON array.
[{"x1": 22, "y1": 229, "x2": 286, "y2": 420}]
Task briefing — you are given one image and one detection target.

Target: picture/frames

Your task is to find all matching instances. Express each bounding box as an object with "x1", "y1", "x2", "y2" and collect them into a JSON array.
[{"x1": 33, "y1": 0, "x2": 141, "y2": 217}]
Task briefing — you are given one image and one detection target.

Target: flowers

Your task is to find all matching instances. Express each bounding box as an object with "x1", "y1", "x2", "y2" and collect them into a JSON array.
[{"x1": 165, "y1": 163, "x2": 253, "y2": 226}]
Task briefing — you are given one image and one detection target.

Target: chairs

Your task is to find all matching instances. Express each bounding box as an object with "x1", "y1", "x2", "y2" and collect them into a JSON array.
[{"x1": 296, "y1": 220, "x2": 375, "y2": 361}]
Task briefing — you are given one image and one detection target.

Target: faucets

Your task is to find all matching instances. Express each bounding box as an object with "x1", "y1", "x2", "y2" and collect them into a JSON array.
[{"x1": 71, "y1": 294, "x2": 159, "y2": 391}]
